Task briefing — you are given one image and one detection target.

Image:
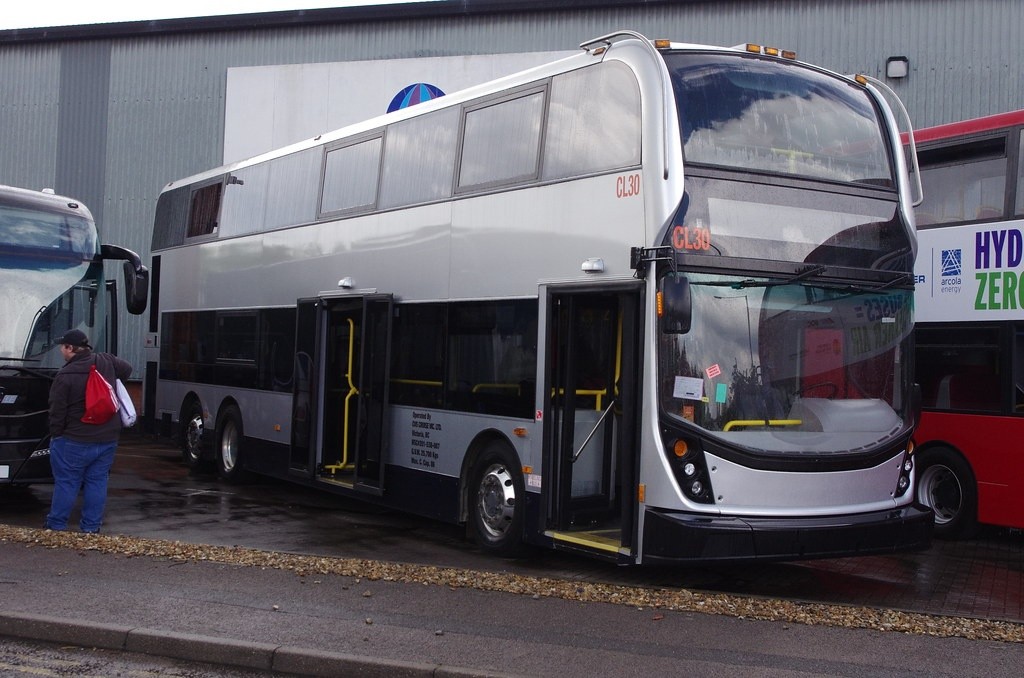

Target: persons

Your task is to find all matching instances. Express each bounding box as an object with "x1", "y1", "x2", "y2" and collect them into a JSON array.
[
  {"x1": 717, "y1": 311, "x2": 798, "y2": 429},
  {"x1": 42, "y1": 329, "x2": 132, "y2": 532}
]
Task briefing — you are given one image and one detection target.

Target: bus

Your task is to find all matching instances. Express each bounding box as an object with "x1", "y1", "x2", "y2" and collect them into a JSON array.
[
  {"x1": 716, "y1": 110, "x2": 1024, "y2": 545},
  {"x1": 0, "y1": 187, "x2": 148, "y2": 492},
  {"x1": 142, "y1": 28, "x2": 936, "y2": 571}
]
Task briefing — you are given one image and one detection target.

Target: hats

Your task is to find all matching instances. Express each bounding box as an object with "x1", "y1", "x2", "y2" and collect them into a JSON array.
[{"x1": 53, "y1": 329, "x2": 89, "y2": 346}]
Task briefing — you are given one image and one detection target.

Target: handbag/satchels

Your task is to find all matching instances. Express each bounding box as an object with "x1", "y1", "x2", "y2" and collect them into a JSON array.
[{"x1": 110, "y1": 352, "x2": 137, "y2": 428}]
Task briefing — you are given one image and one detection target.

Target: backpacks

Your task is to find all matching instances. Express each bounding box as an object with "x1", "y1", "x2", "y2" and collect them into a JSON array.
[{"x1": 81, "y1": 353, "x2": 121, "y2": 424}]
